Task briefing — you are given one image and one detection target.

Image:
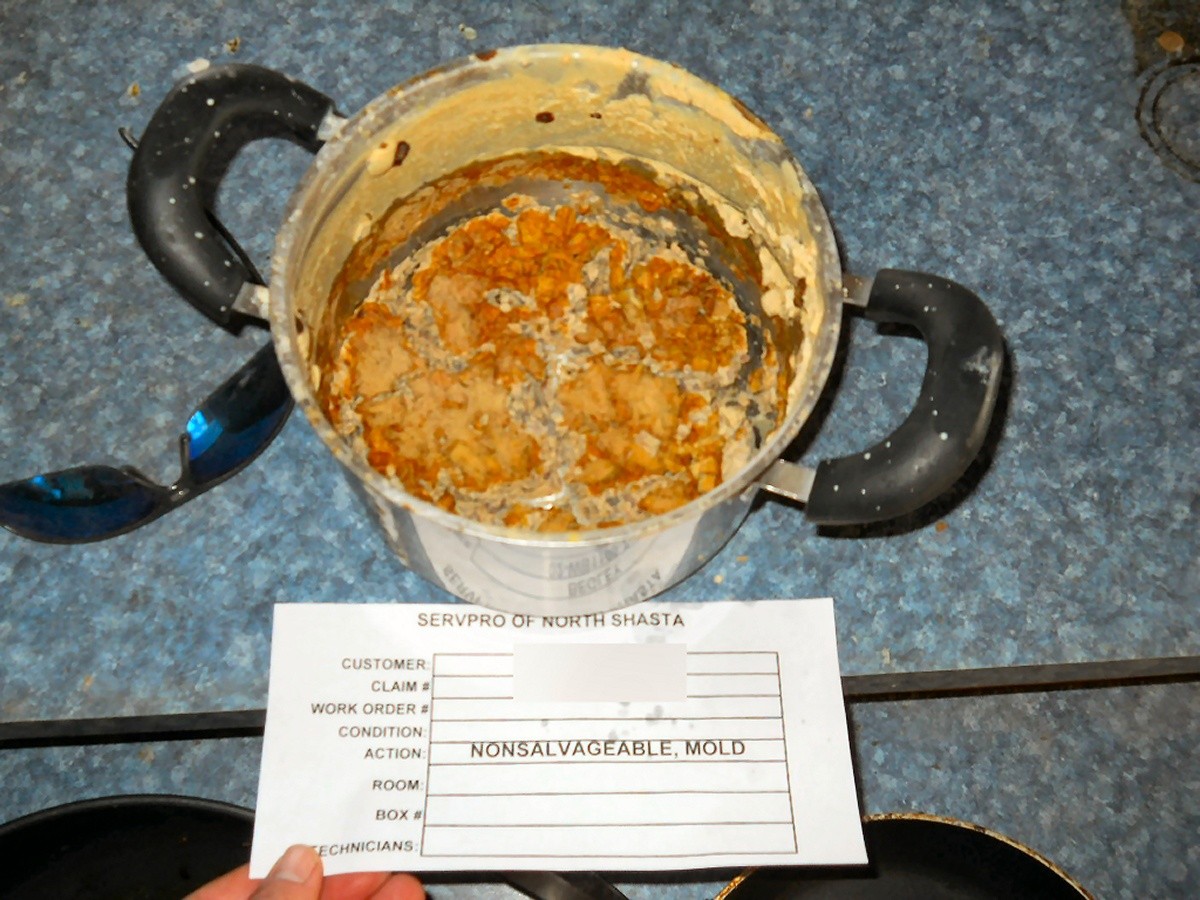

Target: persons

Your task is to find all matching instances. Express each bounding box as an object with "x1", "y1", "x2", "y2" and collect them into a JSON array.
[{"x1": 181, "y1": 844, "x2": 426, "y2": 900}]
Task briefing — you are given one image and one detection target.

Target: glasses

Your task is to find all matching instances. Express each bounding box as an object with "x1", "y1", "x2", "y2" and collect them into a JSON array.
[{"x1": 0, "y1": 126, "x2": 296, "y2": 544}]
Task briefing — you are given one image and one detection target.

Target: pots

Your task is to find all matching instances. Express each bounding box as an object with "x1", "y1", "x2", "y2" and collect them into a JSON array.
[
  {"x1": 124, "y1": 43, "x2": 1000, "y2": 615},
  {"x1": 475, "y1": 776, "x2": 1094, "y2": 900},
  {"x1": 0, "y1": 767, "x2": 437, "y2": 900}
]
